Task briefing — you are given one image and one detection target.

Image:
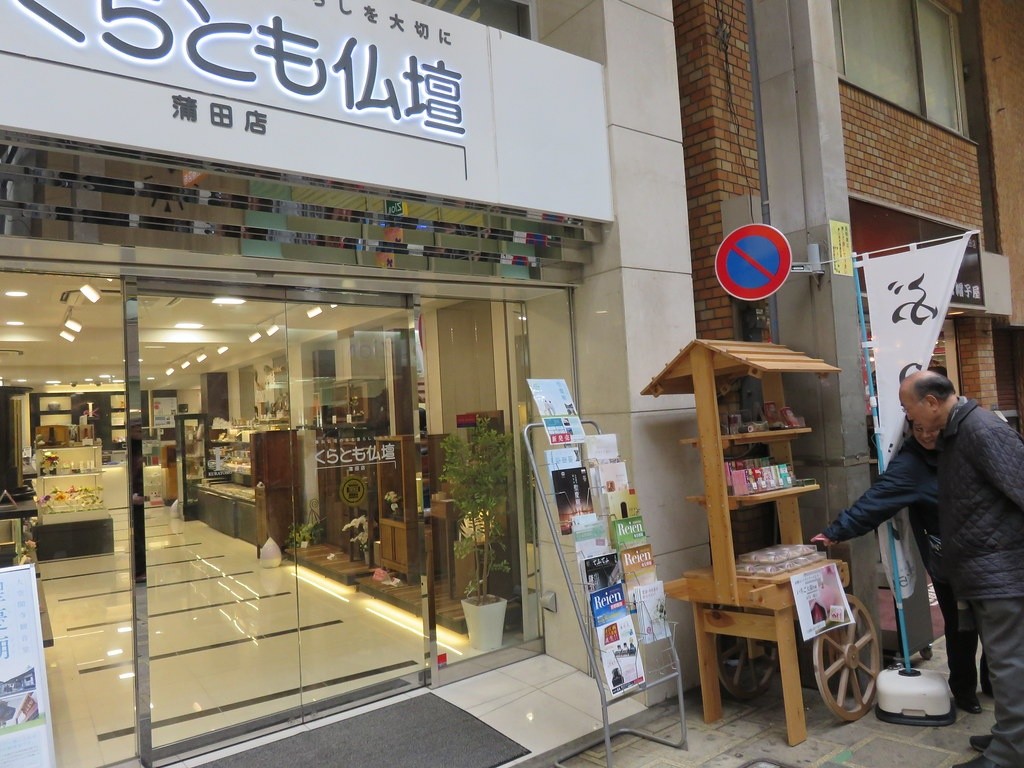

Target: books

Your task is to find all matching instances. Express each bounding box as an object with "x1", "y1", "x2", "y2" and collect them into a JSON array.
[{"x1": 544, "y1": 433, "x2": 671, "y2": 698}]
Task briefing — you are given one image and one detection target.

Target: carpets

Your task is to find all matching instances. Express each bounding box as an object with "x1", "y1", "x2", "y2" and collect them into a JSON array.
[{"x1": 194, "y1": 691, "x2": 531, "y2": 768}]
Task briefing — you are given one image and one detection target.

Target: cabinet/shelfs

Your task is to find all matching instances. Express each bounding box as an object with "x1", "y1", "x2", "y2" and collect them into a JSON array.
[{"x1": 373, "y1": 433, "x2": 458, "y2": 587}]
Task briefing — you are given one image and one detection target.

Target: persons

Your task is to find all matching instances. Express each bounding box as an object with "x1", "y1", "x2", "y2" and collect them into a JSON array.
[
  {"x1": 898, "y1": 371, "x2": 1024, "y2": 768},
  {"x1": 810, "y1": 415, "x2": 993, "y2": 714}
]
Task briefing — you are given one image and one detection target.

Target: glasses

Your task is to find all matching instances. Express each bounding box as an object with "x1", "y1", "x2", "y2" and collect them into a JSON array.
[{"x1": 902, "y1": 397, "x2": 924, "y2": 413}]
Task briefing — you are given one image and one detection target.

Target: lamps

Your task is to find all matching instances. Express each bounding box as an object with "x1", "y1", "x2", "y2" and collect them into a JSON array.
[
  {"x1": 79, "y1": 282, "x2": 102, "y2": 304},
  {"x1": 64, "y1": 317, "x2": 84, "y2": 333},
  {"x1": 60, "y1": 328, "x2": 78, "y2": 342},
  {"x1": 58, "y1": 289, "x2": 80, "y2": 303}
]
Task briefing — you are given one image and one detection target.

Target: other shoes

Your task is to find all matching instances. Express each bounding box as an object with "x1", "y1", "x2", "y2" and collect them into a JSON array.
[
  {"x1": 954, "y1": 694, "x2": 982, "y2": 713},
  {"x1": 982, "y1": 686, "x2": 994, "y2": 698},
  {"x1": 969, "y1": 735, "x2": 994, "y2": 750},
  {"x1": 951, "y1": 756, "x2": 1000, "y2": 768}
]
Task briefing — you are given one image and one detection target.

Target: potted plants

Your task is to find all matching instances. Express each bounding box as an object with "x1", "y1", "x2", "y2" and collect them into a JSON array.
[{"x1": 437, "y1": 412, "x2": 535, "y2": 650}]
